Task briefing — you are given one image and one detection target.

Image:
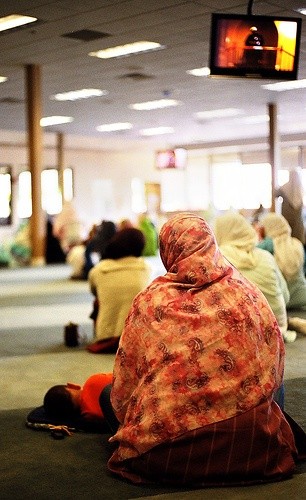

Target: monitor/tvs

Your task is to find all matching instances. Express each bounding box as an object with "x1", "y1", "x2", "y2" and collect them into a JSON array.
[{"x1": 209, "y1": 12, "x2": 302, "y2": 81}]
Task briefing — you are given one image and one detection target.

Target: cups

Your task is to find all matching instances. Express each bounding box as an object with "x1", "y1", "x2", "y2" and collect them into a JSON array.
[{"x1": 65, "y1": 325, "x2": 77, "y2": 347}]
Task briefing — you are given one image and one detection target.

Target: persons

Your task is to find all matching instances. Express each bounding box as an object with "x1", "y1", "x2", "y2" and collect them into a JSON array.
[
  {"x1": 44, "y1": 373, "x2": 114, "y2": 433},
  {"x1": 0, "y1": 167, "x2": 306, "y2": 354},
  {"x1": 99, "y1": 211, "x2": 306, "y2": 487}
]
